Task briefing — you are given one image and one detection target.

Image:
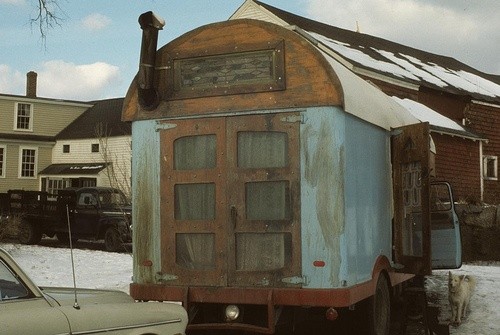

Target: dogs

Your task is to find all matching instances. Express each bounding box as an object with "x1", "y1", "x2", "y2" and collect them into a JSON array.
[{"x1": 448, "y1": 271, "x2": 476, "y2": 323}]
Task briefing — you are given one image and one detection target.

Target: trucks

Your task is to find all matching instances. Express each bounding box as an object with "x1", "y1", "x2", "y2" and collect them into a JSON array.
[
  {"x1": 0, "y1": 187, "x2": 132, "y2": 252},
  {"x1": 121, "y1": 11, "x2": 462, "y2": 335}
]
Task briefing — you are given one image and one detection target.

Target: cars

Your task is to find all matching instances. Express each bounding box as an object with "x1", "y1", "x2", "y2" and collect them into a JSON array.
[{"x1": 0, "y1": 247, "x2": 189, "y2": 335}]
